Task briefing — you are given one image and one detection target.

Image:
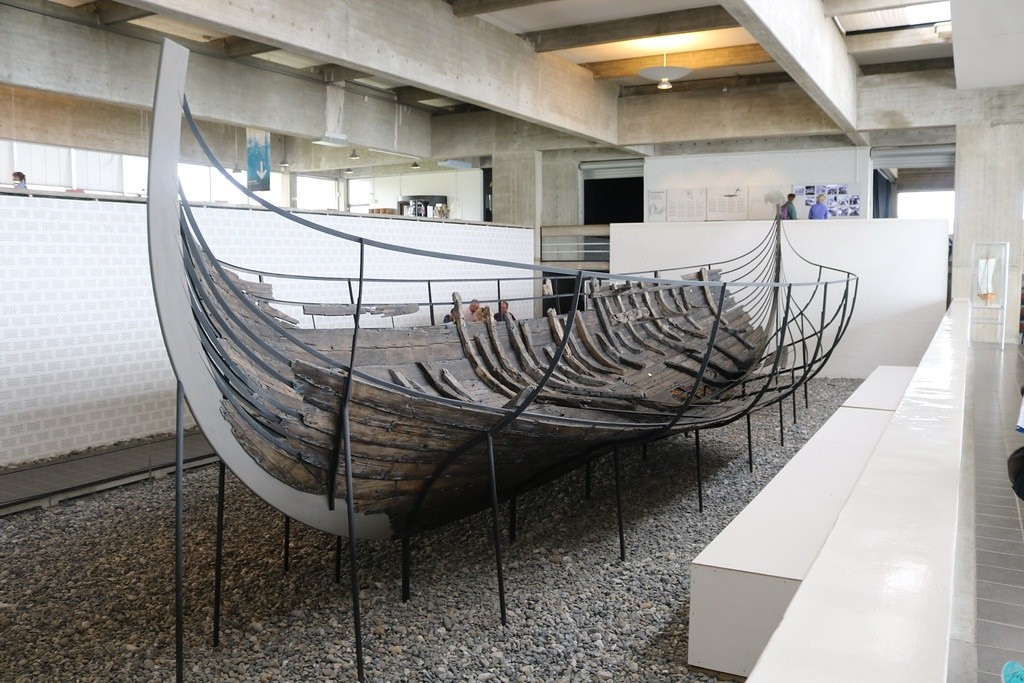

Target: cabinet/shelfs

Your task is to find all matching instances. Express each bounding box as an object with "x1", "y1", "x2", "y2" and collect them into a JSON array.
[{"x1": 968, "y1": 240, "x2": 1010, "y2": 350}]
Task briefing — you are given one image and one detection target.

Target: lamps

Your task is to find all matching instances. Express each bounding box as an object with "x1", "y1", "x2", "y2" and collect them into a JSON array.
[
  {"x1": 411, "y1": 158, "x2": 421, "y2": 170},
  {"x1": 233, "y1": 127, "x2": 241, "y2": 173},
  {"x1": 343, "y1": 149, "x2": 353, "y2": 173},
  {"x1": 279, "y1": 135, "x2": 289, "y2": 166},
  {"x1": 635, "y1": 49, "x2": 693, "y2": 90},
  {"x1": 348, "y1": 149, "x2": 360, "y2": 159}
]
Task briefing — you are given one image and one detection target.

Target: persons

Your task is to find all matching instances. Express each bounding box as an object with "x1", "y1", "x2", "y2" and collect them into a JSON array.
[
  {"x1": 784, "y1": 194, "x2": 797, "y2": 220},
  {"x1": 443, "y1": 298, "x2": 516, "y2": 324},
  {"x1": 808, "y1": 194, "x2": 828, "y2": 219},
  {"x1": 12, "y1": 172, "x2": 26, "y2": 189}
]
